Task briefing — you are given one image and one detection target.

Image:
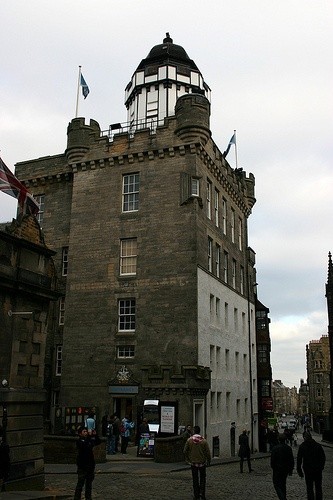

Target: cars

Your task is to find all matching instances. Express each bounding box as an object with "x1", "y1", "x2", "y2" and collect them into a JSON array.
[{"x1": 267, "y1": 417, "x2": 298, "y2": 433}]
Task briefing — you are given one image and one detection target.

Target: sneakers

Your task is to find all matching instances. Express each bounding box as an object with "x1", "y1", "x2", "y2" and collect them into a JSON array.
[{"x1": 185, "y1": 462, "x2": 192, "y2": 465}]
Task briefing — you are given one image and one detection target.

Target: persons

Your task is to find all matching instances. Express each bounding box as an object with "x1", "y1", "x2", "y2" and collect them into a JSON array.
[
  {"x1": 270, "y1": 435, "x2": 295, "y2": 500},
  {"x1": 297, "y1": 431, "x2": 326, "y2": 500},
  {"x1": 85, "y1": 412, "x2": 149, "y2": 455},
  {"x1": 183, "y1": 425, "x2": 212, "y2": 500},
  {"x1": 238, "y1": 429, "x2": 254, "y2": 473},
  {"x1": 261, "y1": 420, "x2": 312, "y2": 446},
  {"x1": 74, "y1": 427, "x2": 102, "y2": 500}
]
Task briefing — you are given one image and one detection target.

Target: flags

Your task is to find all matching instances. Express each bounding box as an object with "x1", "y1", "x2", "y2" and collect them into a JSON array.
[
  {"x1": 223, "y1": 134, "x2": 236, "y2": 159},
  {"x1": 80, "y1": 74, "x2": 90, "y2": 99}
]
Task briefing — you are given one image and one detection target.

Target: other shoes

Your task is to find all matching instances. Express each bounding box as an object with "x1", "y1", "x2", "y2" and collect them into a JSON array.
[
  {"x1": 200, "y1": 493, "x2": 206, "y2": 500},
  {"x1": 108, "y1": 453, "x2": 114, "y2": 455},
  {"x1": 122, "y1": 453, "x2": 128, "y2": 455},
  {"x1": 194, "y1": 496, "x2": 199, "y2": 500},
  {"x1": 112, "y1": 452, "x2": 117, "y2": 455}
]
